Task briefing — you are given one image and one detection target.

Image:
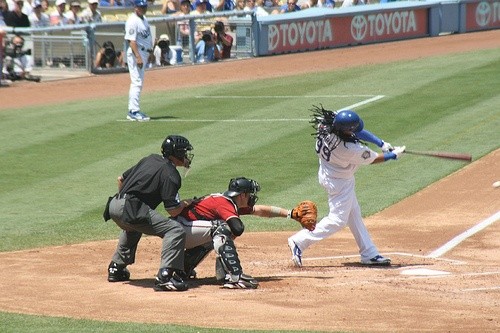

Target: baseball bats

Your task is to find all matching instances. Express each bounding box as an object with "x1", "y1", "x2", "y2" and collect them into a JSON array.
[{"x1": 388, "y1": 148, "x2": 472, "y2": 161}]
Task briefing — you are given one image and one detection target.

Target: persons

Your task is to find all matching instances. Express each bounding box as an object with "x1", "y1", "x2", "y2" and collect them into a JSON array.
[
  {"x1": 125, "y1": 0, "x2": 153, "y2": 122},
  {"x1": 287, "y1": 104, "x2": 406, "y2": 268},
  {"x1": 107, "y1": 136, "x2": 195, "y2": 292},
  {"x1": 0, "y1": 0, "x2": 102, "y2": 74},
  {"x1": 169, "y1": 177, "x2": 317, "y2": 289},
  {"x1": 94, "y1": 22, "x2": 233, "y2": 68},
  {"x1": 162, "y1": 0, "x2": 371, "y2": 49}
]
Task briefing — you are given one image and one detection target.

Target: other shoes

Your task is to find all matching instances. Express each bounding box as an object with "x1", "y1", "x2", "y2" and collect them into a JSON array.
[
  {"x1": 152, "y1": 268, "x2": 188, "y2": 292},
  {"x1": 223, "y1": 273, "x2": 259, "y2": 290},
  {"x1": 107, "y1": 261, "x2": 130, "y2": 282},
  {"x1": 188, "y1": 270, "x2": 196, "y2": 279},
  {"x1": 126, "y1": 110, "x2": 150, "y2": 122}
]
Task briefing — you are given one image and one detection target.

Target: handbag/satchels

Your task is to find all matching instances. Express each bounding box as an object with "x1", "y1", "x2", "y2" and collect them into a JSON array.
[{"x1": 103, "y1": 196, "x2": 113, "y2": 222}]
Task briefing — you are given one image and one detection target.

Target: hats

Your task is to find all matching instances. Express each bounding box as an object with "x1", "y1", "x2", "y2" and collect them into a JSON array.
[
  {"x1": 32, "y1": 1, "x2": 41, "y2": 8},
  {"x1": 14, "y1": 1, "x2": 23, "y2": 6},
  {"x1": 136, "y1": 1, "x2": 147, "y2": 7},
  {"x1": 195, "y1": 0, "x2": 207, "y2": 6},
  {"x1": 71, "y1": 1, "x2": 80, "y2": 6},
  {"x1": 55, "y1": 0, "x2": 66, "y2": 6},
  {"x1": 87, "y1": 0, "x2": 98, "y2": 4},
  {"x1": 159, "y1": 34, "x2": 170, "y2": 42},
  {"x1": 180, "y1": 0, "x2": 190, "y2": 5}
]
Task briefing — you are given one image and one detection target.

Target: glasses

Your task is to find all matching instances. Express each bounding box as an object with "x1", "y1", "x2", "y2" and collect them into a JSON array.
[{"x1": 287, "y1": 2, "x2": 295, "y2": 5}]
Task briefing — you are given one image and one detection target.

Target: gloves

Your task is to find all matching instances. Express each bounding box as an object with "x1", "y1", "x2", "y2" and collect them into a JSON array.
[
  {"x1": 382, "y1": 142, "x2": 392, "y2": 153},
  {"x1": 392, "y1": 145, "x2": 406, "y2": 158}
]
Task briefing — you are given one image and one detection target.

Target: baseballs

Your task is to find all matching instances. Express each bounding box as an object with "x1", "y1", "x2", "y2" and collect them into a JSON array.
[{"x1": 492, "y1": 181, "x2": 500, "y2": 188}]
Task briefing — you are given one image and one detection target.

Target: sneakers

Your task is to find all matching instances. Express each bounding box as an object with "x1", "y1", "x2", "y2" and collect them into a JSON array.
[
  {"x1": 361, "y1": 255, "x2": 391, "y2": 265},
  {"x1": 288, "y1": 237, "x2": 303, "y2": 268}
]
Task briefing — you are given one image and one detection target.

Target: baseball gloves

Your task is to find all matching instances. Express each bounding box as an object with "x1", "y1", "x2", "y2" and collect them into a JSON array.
[{"x1": 289, "y1": 200, "x2": 317, "y2": 232}]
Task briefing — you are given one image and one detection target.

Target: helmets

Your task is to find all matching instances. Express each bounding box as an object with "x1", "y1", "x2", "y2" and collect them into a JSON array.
[
  {"x1": 160, "y1": 134, "x2": 195, "y2": 168},
  {"x1": 223, "y1": 177, "x2": 260, "y2": 197},
  {"x1": 332, "y1": 110, "x2": 363, "y2": 133}
]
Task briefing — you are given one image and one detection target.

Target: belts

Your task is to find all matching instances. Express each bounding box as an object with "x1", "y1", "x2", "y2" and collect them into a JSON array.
[
  {"x1": 116, "y1": 194, "x2": 126, "y2": 199},
  {"x1": 142, "y1": 48, "x2": 152, "y2": 52}
]
